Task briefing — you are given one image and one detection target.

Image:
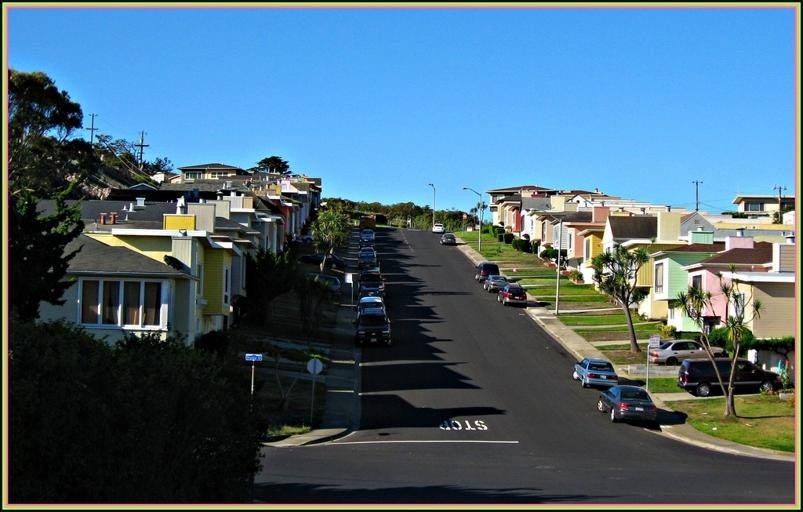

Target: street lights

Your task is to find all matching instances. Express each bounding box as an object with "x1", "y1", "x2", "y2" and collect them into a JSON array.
[
  {"x1": 427, "y1": 183, "x2": 436, "y2": 225},
  {"x1": 462, "y1": 187, "x2": 482, "y2": 251},
  {"x1": 524, "y1": 208, "x2": 562, "y2": 316}
]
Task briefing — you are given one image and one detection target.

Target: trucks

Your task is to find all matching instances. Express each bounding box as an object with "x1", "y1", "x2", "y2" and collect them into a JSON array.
[{"x1": 358, "y1": 213, "x2": 376, "y2": 231}]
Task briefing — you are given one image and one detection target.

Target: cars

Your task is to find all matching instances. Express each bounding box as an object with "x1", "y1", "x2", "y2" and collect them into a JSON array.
[
  {"x1": 572, "y1": 356, "x2": 657, "y2": 423},
  {"x1": 299, "y1": 253, "x2": 348, "y2": 269},
  {"x1": 432, "y1": 223, "x2": 445, "y2": 234},
  {"x1": 357, "y1": 228, "x2": 393, "y2": 345},
  {"x1": 299, "y1": 230, "x2": 316, "y2": 243},
  {"x1": 649, "y1": 339, "x2": 728, "y2": 367},
  {"x1": 439, "y1": 233, "x2": 456, "y2": 246},
  {"x1": 474, "y1": 262, "x2": 527, "y2": 308},
  {"x1": 306, "y1": 272, "x2": 344, "y2": 300}
]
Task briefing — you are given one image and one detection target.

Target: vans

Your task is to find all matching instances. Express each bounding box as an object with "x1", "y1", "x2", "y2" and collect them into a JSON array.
[{"x1": 676, "y1": 357, "x2": 783, "y2": 397}]
